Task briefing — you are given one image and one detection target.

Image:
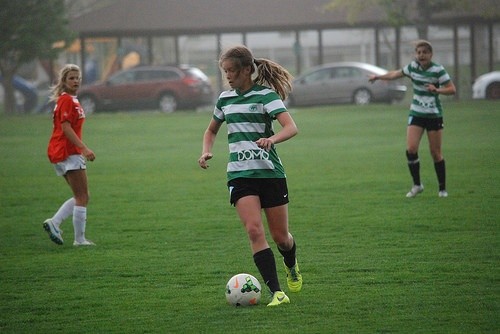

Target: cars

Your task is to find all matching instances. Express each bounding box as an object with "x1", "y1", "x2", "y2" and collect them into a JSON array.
[
  {"x1": 282, "y1": 61, "x2": 408, "y2": 107},
  {"x1": 470, "y1": 70, "x2": 500, "y2": 100}
]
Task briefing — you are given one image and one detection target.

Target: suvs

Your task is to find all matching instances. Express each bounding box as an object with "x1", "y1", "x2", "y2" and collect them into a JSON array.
[{"x1": 73, "y1": 63, "x2": 214, "y2": 113}]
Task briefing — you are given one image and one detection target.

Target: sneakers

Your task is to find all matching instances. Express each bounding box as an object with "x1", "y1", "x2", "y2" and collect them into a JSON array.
[
  {"x1": 283, "y1": 260, "x2": 303, "y2": 292},
  {"x1": 267, "y1": 292, "x2": 290, "y2": 306},
  {"x1": 43, "y1": 219, "x2": 63, "y2": 245},
  {"x1": 73, "y1": 239, "x2": 97, "y2": 246},
  {"x1": 439, "y1": 191, "x2": 448, "y2": 197},
  {"x1": 406, "y1": 184, "x2": 423, "y2": 198}
]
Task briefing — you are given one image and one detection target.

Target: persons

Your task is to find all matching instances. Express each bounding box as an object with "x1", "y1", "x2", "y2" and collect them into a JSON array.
[
  {"x1": 368, "y1": 42, "x2": 458, "y2": 197},
  {"x1": 200, "y1": 46, "x2": 303, "y2": 308},
  {"x1": 39, "y1": 64, "x2": 98, "y2": 246}
]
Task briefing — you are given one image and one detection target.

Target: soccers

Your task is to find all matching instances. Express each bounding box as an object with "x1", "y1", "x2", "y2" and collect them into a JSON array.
[{"x1": 226, "y1": 273, "x2": 262, "y2": 306}]
